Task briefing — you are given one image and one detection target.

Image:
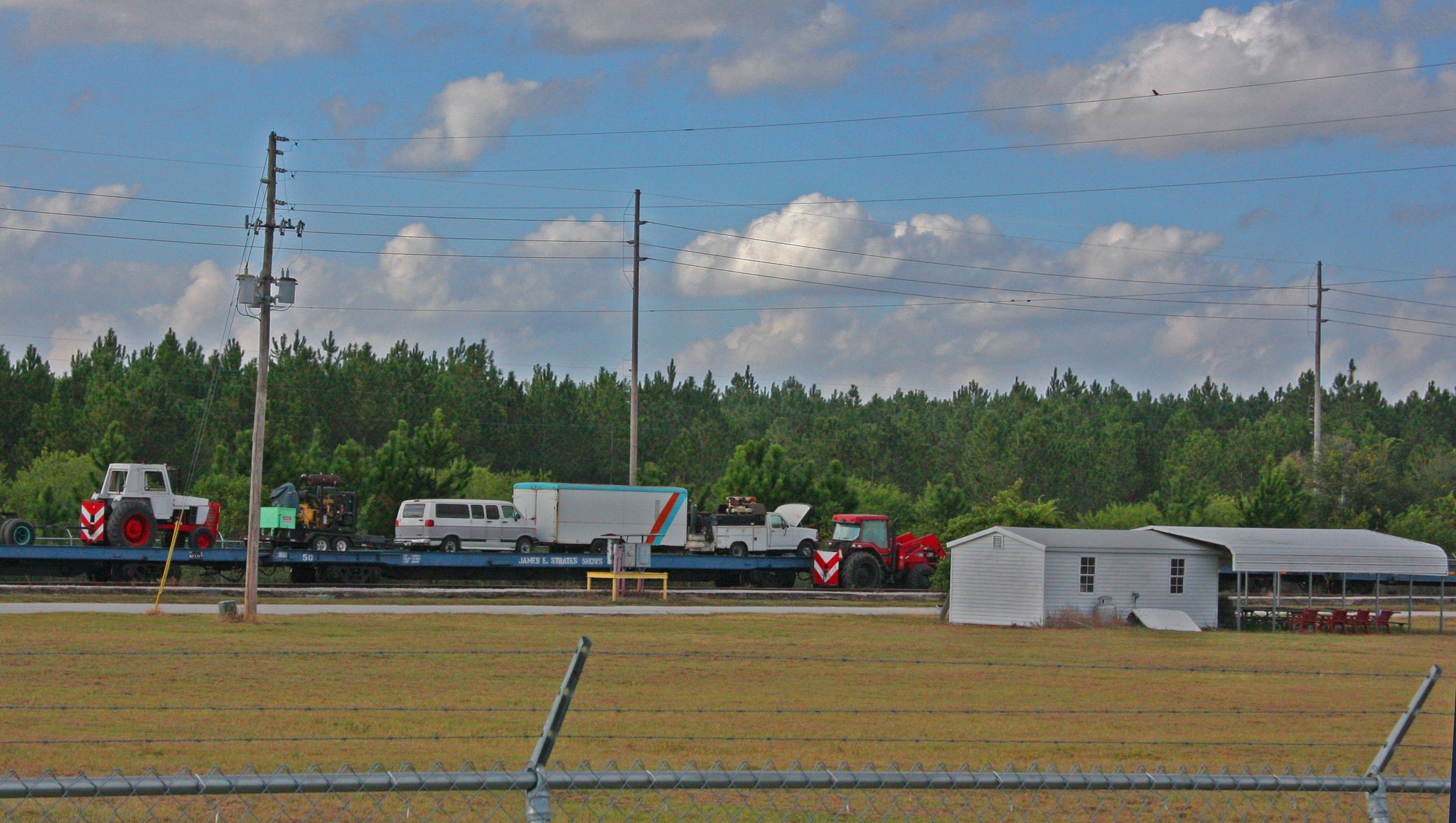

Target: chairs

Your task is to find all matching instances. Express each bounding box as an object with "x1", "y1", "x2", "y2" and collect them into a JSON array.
[
  {"x1": 1317, "y1": 609, "x2": 1346, "y2": 635},
  {"x1": 1288, "y1": 607, "x2": 1320, "y2": 633},
  {"x1": 1346, "y1": 609, "x2": 1371, "y2": 635},
  {"x1": 1370, "y1": 609, "x2": 1394, "y2": 634}
]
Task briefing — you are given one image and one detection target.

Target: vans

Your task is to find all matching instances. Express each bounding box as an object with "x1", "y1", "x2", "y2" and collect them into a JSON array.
[{"x1": 393, "y1": 498, "x2": 540, "y2": 554}]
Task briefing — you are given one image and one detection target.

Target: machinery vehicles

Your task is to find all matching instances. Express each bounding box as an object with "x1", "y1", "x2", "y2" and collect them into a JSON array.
[
  {"x1": 238, "y1": 468, "x2": 385, "y2": 552},
  {"x1": 819, "y1": 513, "x2": 947, "y2": 590},
  {"x1": 79, "y1": 462, "x2": 222, "y2": 548}
]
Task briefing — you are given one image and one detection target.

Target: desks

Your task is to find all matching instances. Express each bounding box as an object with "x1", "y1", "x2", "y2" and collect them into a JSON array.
[{"x1": 1238, "y1": 606, "x2": 1402, "y2": 632}]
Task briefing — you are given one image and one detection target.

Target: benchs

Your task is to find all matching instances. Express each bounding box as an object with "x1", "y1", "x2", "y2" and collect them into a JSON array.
[{"x1": 1389, "y1": 622, "x2": 1407, "y2": 631}]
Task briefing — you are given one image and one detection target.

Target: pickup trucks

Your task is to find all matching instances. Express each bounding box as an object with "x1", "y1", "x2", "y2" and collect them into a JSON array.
[{"x1": 683, "y1": 503, "x2": 822, "y2": 558}]
